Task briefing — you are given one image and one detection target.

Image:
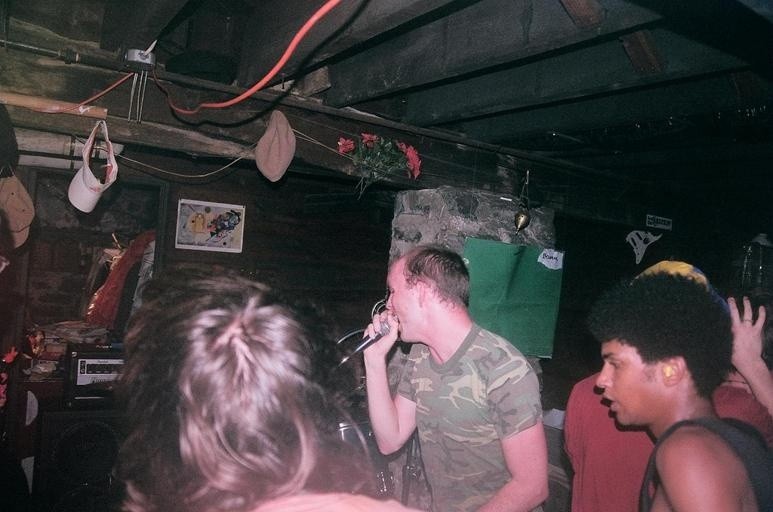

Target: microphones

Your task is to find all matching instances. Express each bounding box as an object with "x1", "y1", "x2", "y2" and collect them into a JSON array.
[{"x1": 337, "y1": 321, "x2": 391, "y2": 368}]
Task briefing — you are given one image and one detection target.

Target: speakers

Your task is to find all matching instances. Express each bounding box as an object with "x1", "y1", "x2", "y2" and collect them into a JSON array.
[{"x1": 25, "y1": 410, "x2": 131, "y2": 512}]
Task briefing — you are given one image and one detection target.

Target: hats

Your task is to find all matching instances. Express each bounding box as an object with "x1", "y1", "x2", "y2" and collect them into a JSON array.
[
  {"x1": 0, "y1": 164, "x2": 36, "y2": 250},
  {"x1": 67, "y1": 120, "x2": 118, "y2": 214}
]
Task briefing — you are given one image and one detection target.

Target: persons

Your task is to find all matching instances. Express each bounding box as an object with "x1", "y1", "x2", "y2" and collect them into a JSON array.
[
  {"x1": 109, "y1": 263, "x2": 422, "y2": 512},
  {"x1": 563, "y1": 260, "x2": 773, "y2": 512},
  {"x1": 362, "y1": 245, "x2": 550, "y2": 512}
]
